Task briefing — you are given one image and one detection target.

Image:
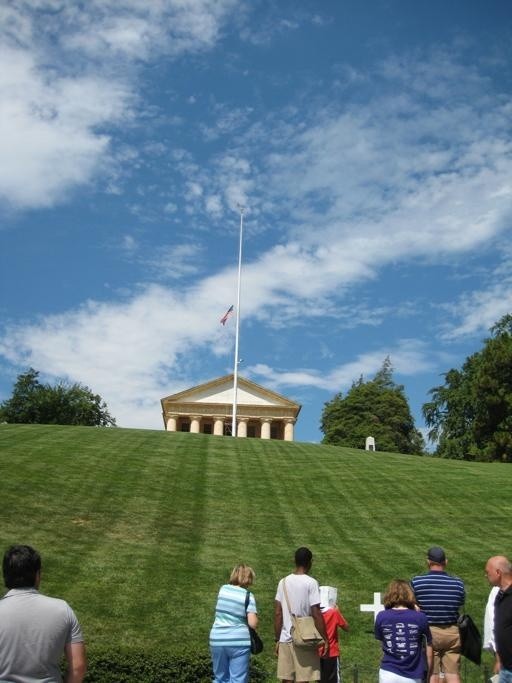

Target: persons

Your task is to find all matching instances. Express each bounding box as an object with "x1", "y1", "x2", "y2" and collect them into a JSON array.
[
  {"x1": 376, "y1": 578, "x2": 434, "y2": 682},
  {"x1": 410, "y1": 546, "x2": 465, "y2": 683},
  {"x1": 485, "y1": 555, "x2": 512, "y2": 683},
  {"x1": 272, "y1": 546, "x2": 330, "y2": 682},
  {"x1": 480, "y1": 583, "x2": 501, "y2": 683},
  {"x1": 208, "y1": 563, "x2": 259, "y2": 682},
  {"x1": 0, "y1": 542, "x2": 88, "y2": 682},
  {"x1": 315, "y1": 585, "x2": 350, "y2": 683}
]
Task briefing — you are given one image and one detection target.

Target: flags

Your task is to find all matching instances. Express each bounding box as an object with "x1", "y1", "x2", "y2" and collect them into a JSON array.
[{"x1": 221, "y1": 303, "x2": 237, "y2": 325}]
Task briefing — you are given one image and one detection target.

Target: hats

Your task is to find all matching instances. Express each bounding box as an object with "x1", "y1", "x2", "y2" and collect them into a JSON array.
[{"x1": 428, "y1": 547, "x2": 445, "y2": 563}]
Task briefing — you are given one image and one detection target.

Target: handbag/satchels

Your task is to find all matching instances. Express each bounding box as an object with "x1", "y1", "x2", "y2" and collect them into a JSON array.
[
  {"x1": 289, "y1": 616, "x2": 326, "y2": 649},
  {"x1": 249, "y1": 628, "x2": 264, "y2": 655},
  {"x1": 457, "y1": 614, "x2": 481, "y2": 665}
]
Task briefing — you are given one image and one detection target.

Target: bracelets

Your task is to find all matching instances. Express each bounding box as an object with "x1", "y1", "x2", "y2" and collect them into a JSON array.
[{"x1": 273, "y1": 635, "x2": 279, "y2": 642}]
